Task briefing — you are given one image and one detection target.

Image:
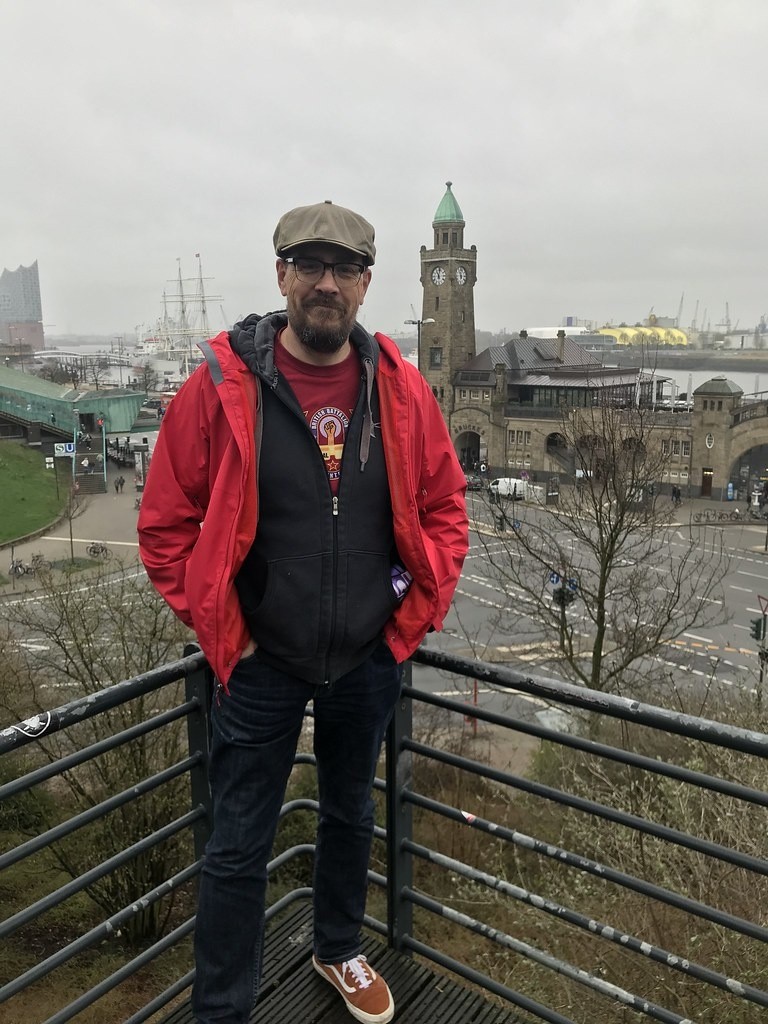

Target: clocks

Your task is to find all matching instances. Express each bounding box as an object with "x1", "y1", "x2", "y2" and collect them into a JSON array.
[
  {"x1": 455, "y1": 266, "x2": 467, "y2": 286},
  {"x1": 431, "y1": 266, "x2": 446, "y2": 286}
]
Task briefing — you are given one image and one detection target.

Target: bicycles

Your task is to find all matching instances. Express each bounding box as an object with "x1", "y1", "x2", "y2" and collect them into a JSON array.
[
  {"x1": 693, "y1": 507, "x2": 768, "y2": 523},
  {"x1": 86, "y1": 541, "x2": 113, "y2": 561},
  {"x1": 7, "y1": 550, "x2": 53, "y2": 579}
]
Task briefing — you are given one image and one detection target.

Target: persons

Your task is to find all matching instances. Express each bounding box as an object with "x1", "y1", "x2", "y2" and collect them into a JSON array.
[
  {"x1": 138, "y1": 197, "x2": 472, "y2": 1023},
  {"x1": 532, "y1": 471, "x2": 537, "y2": 482},
  {"x1": 157, "y1": 406, "x2": 166, "y2": 420},
  {"x1": 114, "y1": 476, "x2": 125, "y2": 493},
  {"x1": 671, "y1": 485, "x2": 684, "y2": 504},
  {"x1": 51, "y1": 413, "x2": 56, "y2": 426},
  {"x1": 747, "y1": 494, "x2": 751, "y2": 511},
  {"x1": 459, "y1": 455, "x2": 480, "y2": 476},
  {"x1": 76, "y1": 417, "x2": 103, "y2": 475}
]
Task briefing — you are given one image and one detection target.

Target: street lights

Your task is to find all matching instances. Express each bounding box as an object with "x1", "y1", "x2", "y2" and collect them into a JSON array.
[{"x1": 404, "y1": 317, "x2": 436, "y2": 376}]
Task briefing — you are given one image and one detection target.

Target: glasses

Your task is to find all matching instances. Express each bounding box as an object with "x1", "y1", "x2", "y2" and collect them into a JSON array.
[{"x1": 285, "y1": 257, "x2": 364, "y2": 289}]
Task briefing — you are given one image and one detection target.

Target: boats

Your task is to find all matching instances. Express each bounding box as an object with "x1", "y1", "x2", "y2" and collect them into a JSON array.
[{"x1": 87, "y1": 253, "x2": 220, "y2": 406}]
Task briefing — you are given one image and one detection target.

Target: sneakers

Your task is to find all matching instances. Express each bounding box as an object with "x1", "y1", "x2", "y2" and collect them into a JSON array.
[{"x1": 312, "y1": 954, "x2": 394, "y2": 1024}]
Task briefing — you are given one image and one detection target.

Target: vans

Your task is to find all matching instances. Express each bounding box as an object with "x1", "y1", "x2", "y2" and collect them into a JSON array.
[{"x1": 487, "y1": 477, "x2": 525, "y2": 502}]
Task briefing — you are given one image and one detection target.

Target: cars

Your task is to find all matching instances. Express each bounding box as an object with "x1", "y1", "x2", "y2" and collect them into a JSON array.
[{"x1": 466, "y1": 474, "x2": 483, "y2": 492}]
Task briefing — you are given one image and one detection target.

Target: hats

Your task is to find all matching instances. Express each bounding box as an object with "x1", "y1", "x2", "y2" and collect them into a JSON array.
[{"x1": 273, "y1": 200, "x2": 376, "y2": 266}]
